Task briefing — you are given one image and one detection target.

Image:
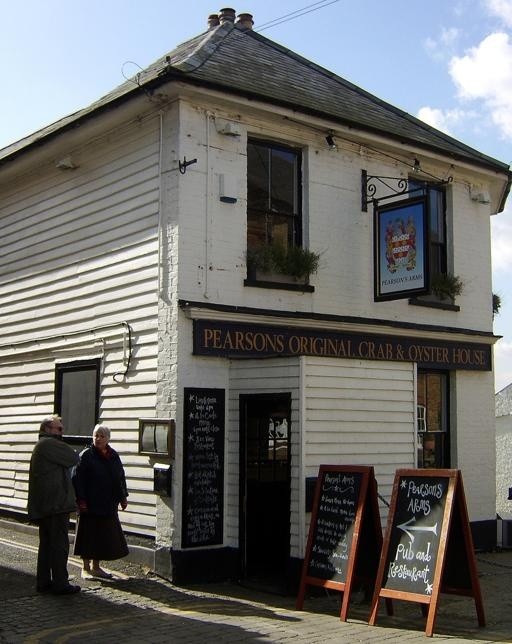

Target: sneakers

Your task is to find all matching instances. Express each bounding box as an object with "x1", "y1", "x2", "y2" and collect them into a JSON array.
[
  {"x1": 92, "y1": 568, "x2": 113, "y2": 578},
  {"x1": 52, "y1": 584, "x2": 81, "y2": 595},
  {"x1": 81, "y1": 568, "x2": 96, "y2": 580}
]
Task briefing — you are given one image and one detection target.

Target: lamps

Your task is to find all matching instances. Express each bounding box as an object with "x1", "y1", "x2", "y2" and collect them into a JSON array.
[
  {"x1": 217, "y1": 122, "x2": 241, "y2": 138},
  {"x1": 411, "y1": 158, "x2": 422, "y2": 172},
  {"x1": 324, "y1": 132, "x2": 337, "y2": 149},
  {"x1": 473, "y1": 192, "x2": 492, "y2": 206},
  {"x1": 54, "y1": 157, "x2": 78, "y2": 170}
]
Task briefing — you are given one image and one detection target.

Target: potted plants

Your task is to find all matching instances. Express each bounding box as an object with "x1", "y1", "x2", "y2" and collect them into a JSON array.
[
  {"x1": 416, "y1": 270, "x2": 465, "y2": 305},
  {"x1": 248, "y1": 243, "x2": 320, "y2": 285}
]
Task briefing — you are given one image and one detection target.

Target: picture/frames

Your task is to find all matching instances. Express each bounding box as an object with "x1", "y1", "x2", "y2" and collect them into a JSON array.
[{"x1": 373, "y1": 193, "x2": 430, "y2": 303}]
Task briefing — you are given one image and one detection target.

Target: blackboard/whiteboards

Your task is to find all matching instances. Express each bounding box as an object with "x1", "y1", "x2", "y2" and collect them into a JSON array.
[
  {"x1": 303, "y1": 465, "x2": 370, "y2": 591},
  {"x1": 183, "y1": 387, "x2": 226, "y2": 548},
  {"x1": 376, "y1": 469, "x2": 459, "y2": 604}
]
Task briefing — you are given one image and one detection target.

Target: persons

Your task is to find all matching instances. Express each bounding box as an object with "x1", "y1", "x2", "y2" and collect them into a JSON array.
[
  {"x1": 70, "y1": 424, "x2": 130, "y2": 582},
  {"x1": 27, "y1": 415, "x2": 82, "y2": 596}
]
